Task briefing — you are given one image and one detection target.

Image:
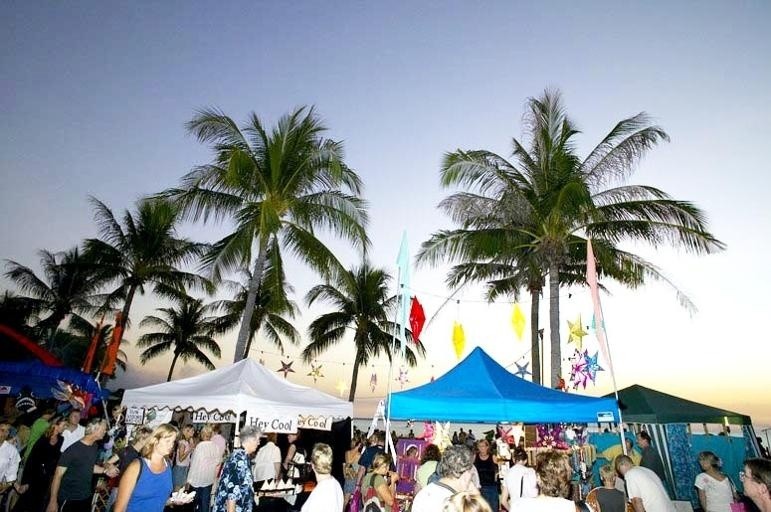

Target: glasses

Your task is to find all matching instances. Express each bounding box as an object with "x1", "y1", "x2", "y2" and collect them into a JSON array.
[{"x1": 742, "y1": 472, "x2": 761, "y2": 485}]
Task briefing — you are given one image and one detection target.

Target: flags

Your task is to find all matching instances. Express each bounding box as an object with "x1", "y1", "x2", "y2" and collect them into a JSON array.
[
  {"x1": 408, "y1": 295, "x2": 425, "y2": 343},
  {"x1": 396, "y1": 231, "x2": 410, "y2": 358},
  {"x1": 585, "y1": 239, "x2": 612, "y2": 367}
]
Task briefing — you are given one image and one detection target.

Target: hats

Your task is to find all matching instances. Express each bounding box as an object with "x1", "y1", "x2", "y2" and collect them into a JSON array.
[{"x1": 405, "y1": 444, "x2": 417, "y2": 452}]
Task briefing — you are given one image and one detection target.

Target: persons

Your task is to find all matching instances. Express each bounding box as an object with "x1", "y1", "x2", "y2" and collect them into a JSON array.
[{"x1": 1, "y1": 407, "x2": 771, "y2": 511}]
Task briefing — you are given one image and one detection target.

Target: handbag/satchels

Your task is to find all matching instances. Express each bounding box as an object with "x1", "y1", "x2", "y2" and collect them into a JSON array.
[
  {"x1": 730, "y1": 502, "x2": 746, "y2": 512},
  {"x1": 349, "y1": 490, "x2": 362, "y2": 512},
  {"x1": 287, "y1": 466, "x2": 301, "y2": 479},
  {"x1": 519, "y1": 473, "x2": 540, "y2": 498},
  {"x1": 363, "y1": 487, "x2": 382, "y2": 512}
]
